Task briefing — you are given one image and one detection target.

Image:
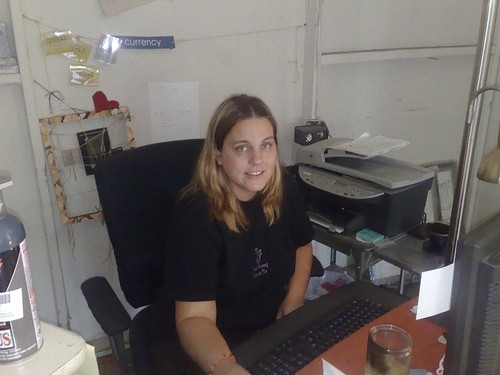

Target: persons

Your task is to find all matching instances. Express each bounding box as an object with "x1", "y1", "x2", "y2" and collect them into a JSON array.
[{"x1": 169, "y1": 93, "x2": 315, "y2": 375}]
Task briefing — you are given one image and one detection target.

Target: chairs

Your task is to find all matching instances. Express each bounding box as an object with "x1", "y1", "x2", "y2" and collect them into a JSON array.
[{"x1": 80, "y1": 138, "x2": 324, "y2": 375}]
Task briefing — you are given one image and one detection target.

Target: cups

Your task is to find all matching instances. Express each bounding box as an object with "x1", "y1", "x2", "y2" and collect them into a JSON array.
[
  {"x1": 364, "y1": 324, "x2": 412, "y2": 375},
  {"x1": 427, "y1": 222, "x2": 450, "y2": 251}
]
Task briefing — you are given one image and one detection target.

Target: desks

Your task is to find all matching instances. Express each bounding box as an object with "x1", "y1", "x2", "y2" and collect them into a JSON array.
[
  {"x1": 0, "y1": 321, "x2": 99, "y2": 375},
  {"x1": 201, "y1": 275, "x2": 482, "y2": 375},
  {"x1": 296, "y1": 219, "x2": 466, "y2": 286}
]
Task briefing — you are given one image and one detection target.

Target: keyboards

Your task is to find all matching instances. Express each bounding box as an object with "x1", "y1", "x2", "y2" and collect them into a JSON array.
[{"x1": 246, "y1": 294, "x2": 391, "y2": 375}]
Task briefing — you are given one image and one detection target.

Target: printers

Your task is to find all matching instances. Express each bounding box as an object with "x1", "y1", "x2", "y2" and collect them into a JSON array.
[{"x1": 296, "y1": 133, "x2": 434, "y2": 239}]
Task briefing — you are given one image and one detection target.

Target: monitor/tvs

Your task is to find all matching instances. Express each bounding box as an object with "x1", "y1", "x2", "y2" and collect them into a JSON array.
[{"x1": 442, "y1": 213, "x2": 500, "y2": 375}]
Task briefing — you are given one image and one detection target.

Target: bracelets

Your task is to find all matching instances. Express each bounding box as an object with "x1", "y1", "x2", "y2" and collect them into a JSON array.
[{"x1": 206, "y1": 352, "x2": 237, "y2": 375}]
